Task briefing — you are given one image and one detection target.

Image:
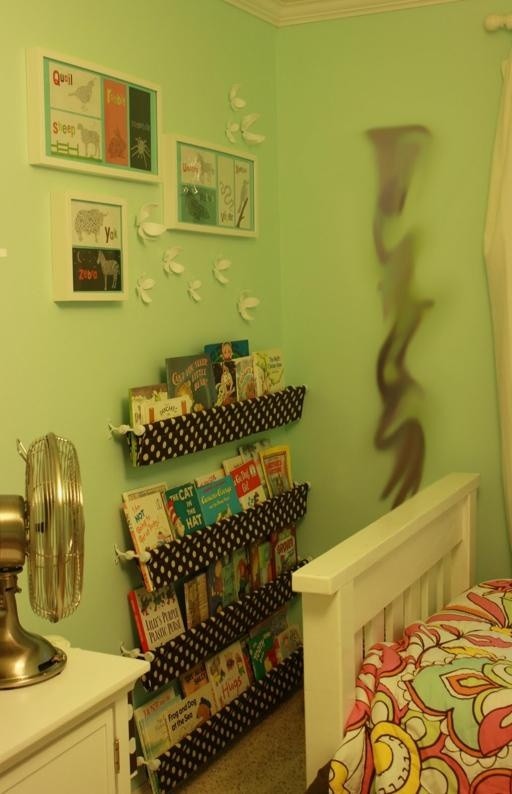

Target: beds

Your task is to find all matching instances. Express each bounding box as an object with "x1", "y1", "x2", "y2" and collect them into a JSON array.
[{"x1": 293, "y1": 472, "x2": 511, "y2": 793}]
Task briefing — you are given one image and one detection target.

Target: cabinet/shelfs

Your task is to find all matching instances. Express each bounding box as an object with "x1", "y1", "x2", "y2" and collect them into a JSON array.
[{"x1": 1, "y1": 646, "x2": 146, "y2": 794}]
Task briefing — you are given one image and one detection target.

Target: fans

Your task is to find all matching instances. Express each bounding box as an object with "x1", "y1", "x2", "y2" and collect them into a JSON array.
[{"x1": 0, "y1": 431, "x2": 86, "y2": 689}]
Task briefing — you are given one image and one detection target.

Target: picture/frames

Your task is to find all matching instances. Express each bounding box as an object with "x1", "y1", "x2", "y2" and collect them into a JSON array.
[
  {"x1": 160, "y1": 132, "x2": 257, "y2": 238},
  {"x1": 45, "y1": 189, "x2": 126, "y2": 305},
  {"x1": 25, "y1": 47, "x2": 166, "y2": 186}
]
